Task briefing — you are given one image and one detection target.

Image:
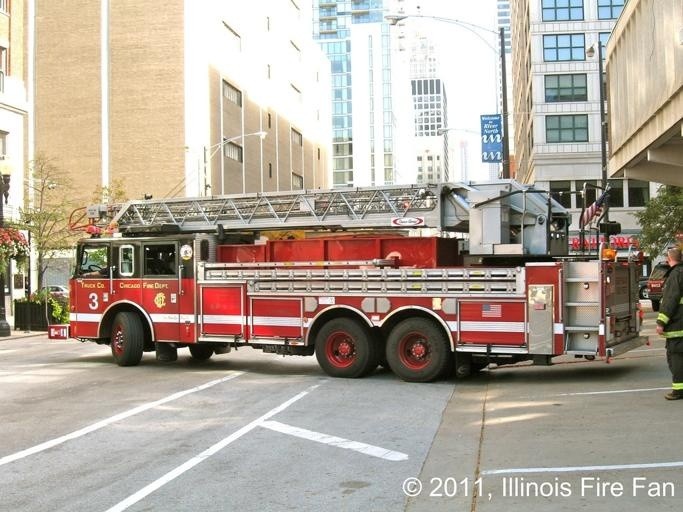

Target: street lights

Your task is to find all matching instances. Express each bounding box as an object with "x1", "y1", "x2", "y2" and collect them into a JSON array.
[
  {"x1": 385, "y1": 12, "x2": 508, "y2": 182},
  {"x1": 0, "y1": 159, "x2": 12, "y2": 335},
  {"x1": 587, "y1": 39, "x2": 609, "y2": 242},
  {"x1": 204, "y1": 131, "x2": 268, "y2": 197}
]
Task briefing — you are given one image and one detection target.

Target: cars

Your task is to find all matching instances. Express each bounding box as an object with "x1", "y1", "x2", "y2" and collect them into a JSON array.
[
  {"x1": 639, "y1": 261, "x2": 673, "y2": 311},
  {"x1": 35, "y1": 285, "x2": 69, "y2": 297}
]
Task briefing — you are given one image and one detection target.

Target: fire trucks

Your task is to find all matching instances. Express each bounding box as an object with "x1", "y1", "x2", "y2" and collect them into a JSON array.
[{"x1": 70, "y1": 179, "x2": 650, "y2": 383}]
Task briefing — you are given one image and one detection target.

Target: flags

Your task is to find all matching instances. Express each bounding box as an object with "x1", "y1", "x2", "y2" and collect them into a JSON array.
[{"x1": 578, "y1": 180, "x2": 610, "y2": 232}]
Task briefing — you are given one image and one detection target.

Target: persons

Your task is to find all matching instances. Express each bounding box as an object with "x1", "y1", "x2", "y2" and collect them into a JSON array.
[{"x1": 654, "y1": 244, "x2": 682, "y2": 402}]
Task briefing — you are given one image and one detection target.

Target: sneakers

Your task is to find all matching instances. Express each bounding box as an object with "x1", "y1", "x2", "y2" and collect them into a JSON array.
[{"x1": 665, "y1": 393, "x2": 683, "y2": 401}]
